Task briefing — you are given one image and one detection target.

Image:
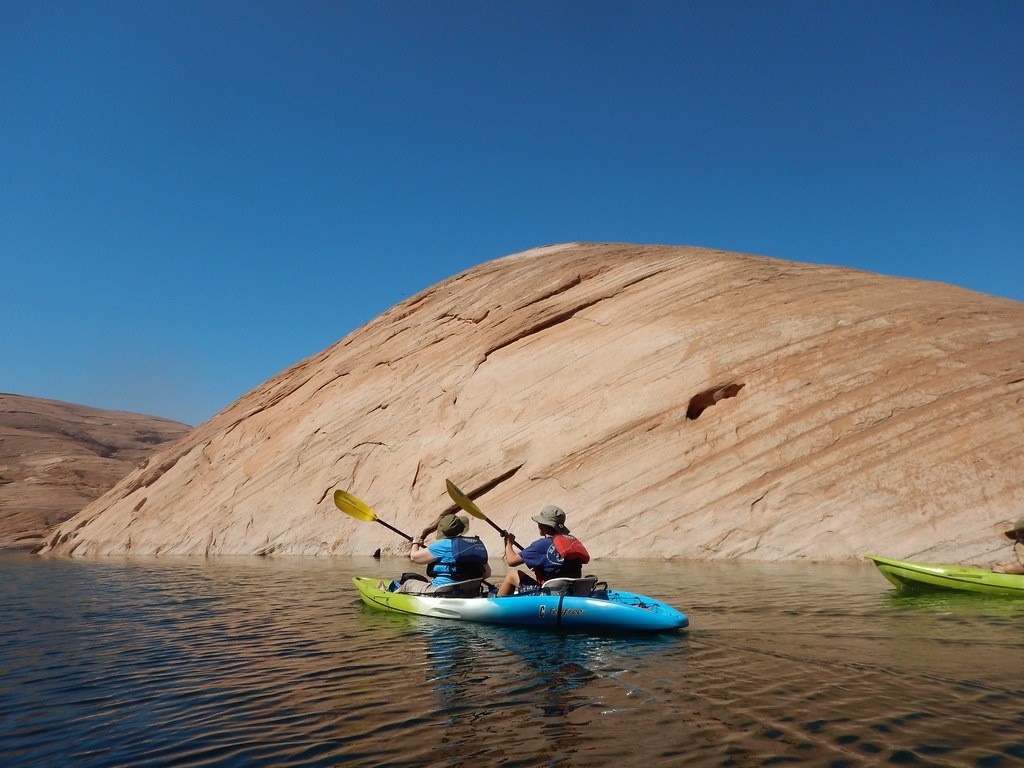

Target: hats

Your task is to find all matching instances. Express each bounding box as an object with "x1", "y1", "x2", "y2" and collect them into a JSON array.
[
  {"x1": 435, "y1": 514, "x2": 469, "y2": 540},
  {"x1": 531, "y1": 505, "x2": 571, "y2": 534}
]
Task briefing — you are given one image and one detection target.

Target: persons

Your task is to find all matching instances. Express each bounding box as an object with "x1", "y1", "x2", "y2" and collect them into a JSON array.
[
  {"x1": 376, "y1": 514, "x2": 492, "y2": 596},
  {"x1": 991, "y1": 516, "x2": 1024, "y2": 574},
  {"x1": 497, "y1": 505, "x2": 589, "y2": 597}
]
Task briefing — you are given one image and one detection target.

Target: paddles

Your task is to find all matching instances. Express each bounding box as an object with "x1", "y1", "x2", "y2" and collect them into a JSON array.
[
  {"x1": 335, "y1": 490, "x2": 416, "y2": 546},
  {"x1": 445, "y1": 478, "x2": 527, "y2": 550}
]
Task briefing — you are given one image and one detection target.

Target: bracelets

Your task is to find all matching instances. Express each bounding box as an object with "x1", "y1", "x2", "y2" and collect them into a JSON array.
[{"x1": 411, "y1": 543, "x2": 419, "y2": 548}]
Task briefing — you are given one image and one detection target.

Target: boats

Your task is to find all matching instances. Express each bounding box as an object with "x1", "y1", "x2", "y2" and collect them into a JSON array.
[
  {"x1": 864, "y1": 554, "x2": 1024, "y2": 598},
  {"x1": 352, "y1": 574, "x2": 690, "y2": 635}
]
